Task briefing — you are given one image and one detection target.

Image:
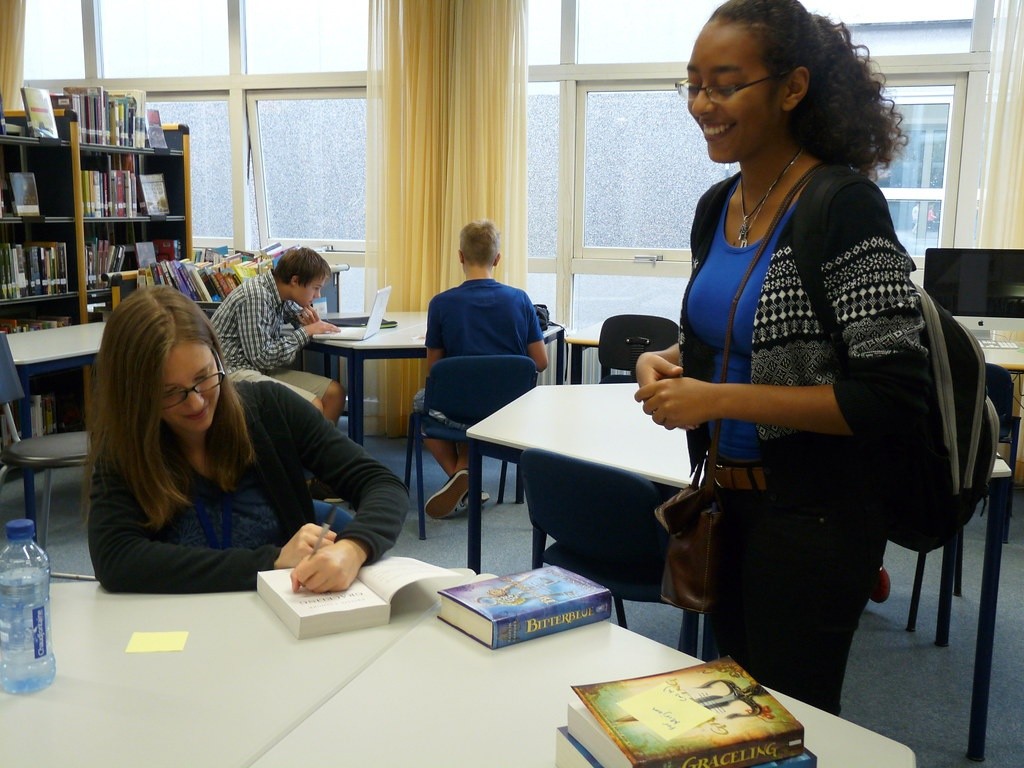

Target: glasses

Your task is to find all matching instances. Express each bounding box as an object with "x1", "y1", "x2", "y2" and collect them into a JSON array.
[
  {"x1": 157, "y1": 346, "x2": 226, "y2": 409},
  {"x1": 675, "y1": 71, "x2": 793, "y2": 102}
]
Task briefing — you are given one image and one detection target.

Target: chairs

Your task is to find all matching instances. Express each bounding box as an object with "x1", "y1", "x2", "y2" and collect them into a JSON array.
[
  {"x1": 980, "y1": 362, "x2": 1021, "y2": 543},
  {"x1": 598, "y1": 315, "x2": 678, "y2": 384},
  {"x1": 403, "y1": 356, "x2": 537, "y2": 540},
  {"x1": 312, "y1": 500, "x2": 354, "y2": 541},
  {"x1": 519, "y1": 448, "x2": 699, "y2": 658},
  {"x1": 0, "y1": 333, "x2": 87, "y2": 549}
]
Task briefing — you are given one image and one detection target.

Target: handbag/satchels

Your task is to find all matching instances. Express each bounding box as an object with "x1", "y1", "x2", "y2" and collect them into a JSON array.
[{"x1": 654, "y1": 485, "x2": 723, "y2": 614}]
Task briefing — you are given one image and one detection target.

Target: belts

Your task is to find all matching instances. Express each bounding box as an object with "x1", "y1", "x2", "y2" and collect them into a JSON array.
[{"x1": 713, "y1": 463, "x2": 766, "y2": 491}]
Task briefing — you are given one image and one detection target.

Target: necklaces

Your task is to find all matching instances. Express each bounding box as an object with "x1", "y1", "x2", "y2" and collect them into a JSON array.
[{"x1": 737, "y1": 146, "x2": 806, "y2": 249}]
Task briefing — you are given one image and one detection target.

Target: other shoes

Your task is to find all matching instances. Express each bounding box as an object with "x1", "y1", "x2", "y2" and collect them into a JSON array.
[
  {"x1": 869, "y1": 564, "x2": 890, "y2": 603},
  {"x1": 463, "y1": 491, "x2": 490, "y2": 508},
  {"x1": 424, "y1": 469, "x2": 469, "y2": 519}
]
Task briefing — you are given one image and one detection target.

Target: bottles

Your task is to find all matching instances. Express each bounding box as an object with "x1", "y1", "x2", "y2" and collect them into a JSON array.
[{"x1": 0, "y1": 518, "x2": 56, "y2": 695}]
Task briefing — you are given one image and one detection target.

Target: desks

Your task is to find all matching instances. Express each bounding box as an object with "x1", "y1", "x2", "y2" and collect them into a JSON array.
[
  {"x1": 278, "y1": 311, "x2": 564, "y2": 503},
  {"x1": 0, "y1": 580, "x2": 916, "y2": 768},
  {"x1": 563, "y1": 322, "x2": 603, "y2": 384},
  {"x1": 6, "y1": 321, "x2": 107, "y2": 564},
  {"x1": 466, "y1": 382, "x2": 1011, "y2": 761},
  {"x1": 953, "y1": 330, "x2": 1024, "y2": 488}
]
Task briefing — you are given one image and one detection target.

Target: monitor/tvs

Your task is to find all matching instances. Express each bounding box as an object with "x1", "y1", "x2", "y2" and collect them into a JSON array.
[{"x1": 924, "y1": 248, "x2": 1024, "y2": 340}]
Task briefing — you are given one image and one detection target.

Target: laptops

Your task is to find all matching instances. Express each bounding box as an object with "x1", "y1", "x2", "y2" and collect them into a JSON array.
[{"x1": 312, "y1": 286, "x2": 391, "y2": 340}]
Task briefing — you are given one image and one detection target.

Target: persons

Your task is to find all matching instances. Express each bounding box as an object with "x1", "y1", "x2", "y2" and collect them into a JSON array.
[
  {"x1": 209, "y1": 248, "x2": 347, "y2": 430},
  {"x1": 418, "y1": 222, "x2": 548, "y2": 521},
  {"x1": 634, "y1": 0, "x2": 931, "y2": 718},
  {"x1": 80, "y1": 285, "x2": 410, "y2": 594}
]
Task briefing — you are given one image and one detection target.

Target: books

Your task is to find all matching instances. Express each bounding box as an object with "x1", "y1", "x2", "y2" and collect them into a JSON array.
[
  {"x1": 436, "y1": 565, "x2": 613, "y2": 650},
  {"x1": 555, "y1": 654, "x2": 818, "y2": 768},
  {"x1": 0, "y1": 85, "x2": 300, "y2": 448},
  {"x1": 256, "y1": 557, "x2": 464, "y2": 640}
]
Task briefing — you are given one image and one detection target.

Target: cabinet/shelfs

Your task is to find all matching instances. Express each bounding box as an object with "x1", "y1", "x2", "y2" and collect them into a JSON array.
[{"x1": 0, "y1": 109, "x2": 194, "y2": 488}]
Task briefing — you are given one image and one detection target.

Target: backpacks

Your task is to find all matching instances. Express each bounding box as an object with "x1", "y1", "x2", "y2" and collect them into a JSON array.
[
  {"x1": 791, "y1": 166, "x2": 1001, "y2": 553},
  {"x1": 532, "y1": 304, "x2": 568, "y2": 380}
]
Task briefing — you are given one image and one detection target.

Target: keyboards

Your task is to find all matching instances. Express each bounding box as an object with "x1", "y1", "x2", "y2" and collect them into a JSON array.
[{"x1": 978, "y1": 340, "x2": 1019, "y2": 349}]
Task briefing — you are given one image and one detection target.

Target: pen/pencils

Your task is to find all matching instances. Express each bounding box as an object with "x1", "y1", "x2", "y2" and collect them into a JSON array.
[{"x1": 297, "y1": 505, "x2": 337, "y2": 591}]
